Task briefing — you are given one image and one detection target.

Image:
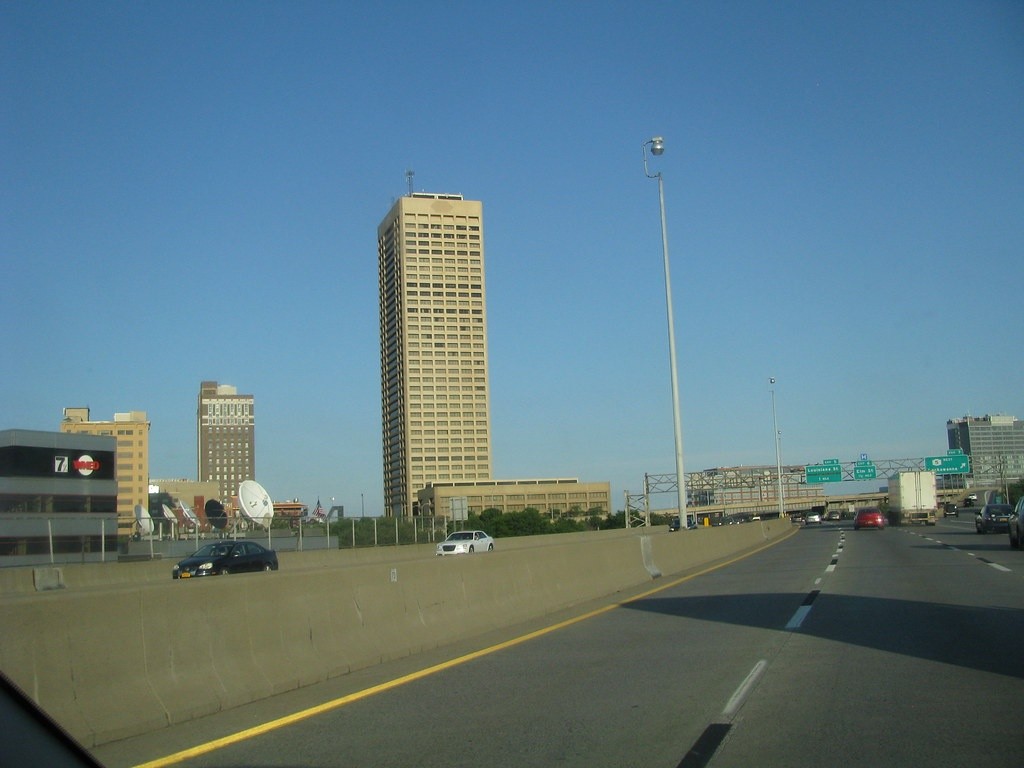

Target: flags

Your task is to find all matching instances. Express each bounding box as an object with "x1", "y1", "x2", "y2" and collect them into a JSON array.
[{"x1": 312, "y1": 499, "x2": 327, "y2": 521}]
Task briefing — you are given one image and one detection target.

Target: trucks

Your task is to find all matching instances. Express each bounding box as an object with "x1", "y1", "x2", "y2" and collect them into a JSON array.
[{"x1": 884, "y1": 470, "x2": 938, "y2": 526}]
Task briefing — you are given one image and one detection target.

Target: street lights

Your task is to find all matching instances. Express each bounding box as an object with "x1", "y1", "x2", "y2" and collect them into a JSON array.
[
  {"x1": 777, "y1": 430, "x2": 788, "y2": 516},
  {"x1": 639, "y1": 136, "x2": 690, "y2": 526},
  {"x1": 765, "y1": 375, "x2": 785, "y2": 519}
]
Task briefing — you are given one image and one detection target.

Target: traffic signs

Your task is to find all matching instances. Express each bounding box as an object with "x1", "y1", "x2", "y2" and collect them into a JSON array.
[
  {"x1": 805, "y1": 465, "x2": 842, "y2": 483},
  {"x1": 924, "y1": 456, "x2": 969, "y2": 474},
  {"x1": 853, "y1": 466, "x2": 877, "y2": 480}
]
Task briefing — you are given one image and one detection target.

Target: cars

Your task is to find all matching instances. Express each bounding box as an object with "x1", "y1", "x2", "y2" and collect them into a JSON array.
[
  {"x1": 827, "y1": 511, "x2": 841, "y2": 521},
  {"x1": 963, "y1": 498, "x2": 974, "y2": 508},
  {"x1": 975, "y1": 504, "x2": 1014, "y2": 534},
  {"x1": 943, "y1": 504, "x2": 959, "y2": 518},
  {"x1": 172, "y1": 541, "x2": 280, "y2": 579},
  {"x1": 668, "y1": 517, "x2": 698, "y2": 532},
  {"x1": 1007, "y1": 496, "x2": 1024, "y2": 550},
  {"x1": 853, "y1": 506, "x2": 884, "y2": 530},
  {"x1": 968, "y1": 492, "x2": 978, "y2": 501},
  {"x1": 789, "y1": 514, "x2": 802, "y2": 522},
  {"x1": 435, "y1": 530, "x2": 496, "y2": 556},
  {"x1": 802, "y1": 512, "x2": 822, "y2": 525},
  {"x1": 717, "y1": 516, "x2": 745, "y2": 525}
]
